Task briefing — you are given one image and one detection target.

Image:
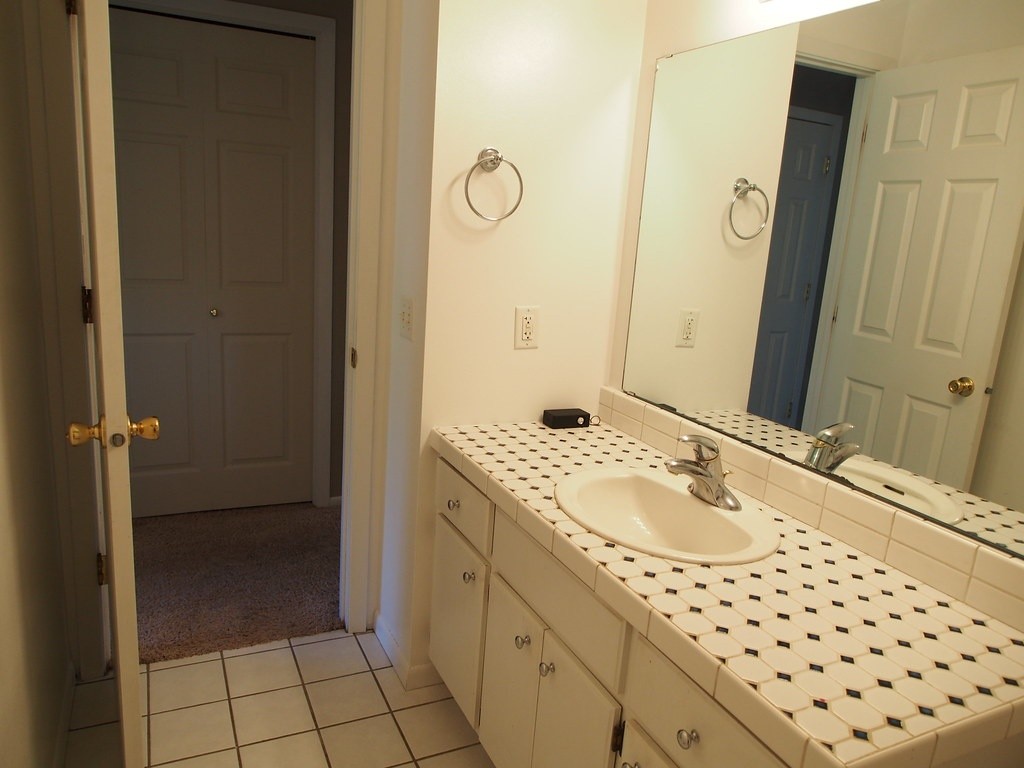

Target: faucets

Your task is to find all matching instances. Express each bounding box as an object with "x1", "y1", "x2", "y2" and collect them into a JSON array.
[
  {"x1": 662, "y1": 433, "x2": 743, "y2": 511},
  {"x1": 800, "y1": 421, "x2": 861, "y2": 475}
]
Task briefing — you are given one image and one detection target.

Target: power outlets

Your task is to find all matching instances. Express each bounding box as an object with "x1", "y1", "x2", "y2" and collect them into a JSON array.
[
  {"x1": 673, "y1": 308, "x2": 700, "y2": 348},
  {"x1": 513, "y1": 306, "x2": 539, "y2": 350}
]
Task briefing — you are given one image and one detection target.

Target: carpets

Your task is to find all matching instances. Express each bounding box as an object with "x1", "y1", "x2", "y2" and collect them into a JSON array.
[{"x1": 133, "y1": 500, "x2": 344, "y2": 664}]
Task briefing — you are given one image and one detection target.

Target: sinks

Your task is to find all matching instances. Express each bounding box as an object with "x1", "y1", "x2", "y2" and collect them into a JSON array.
[
  {"x1": 785, "y1": 451, "x2": 965, "y2": 526},
  {"x1": 554, "y1": 465, "x2": 782, "y2": 566}
]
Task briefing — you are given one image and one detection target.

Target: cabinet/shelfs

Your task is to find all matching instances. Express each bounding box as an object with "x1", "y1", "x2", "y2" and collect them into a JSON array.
[{"x1": 424, "y1": 456, "x2": 790, "y2": 768}]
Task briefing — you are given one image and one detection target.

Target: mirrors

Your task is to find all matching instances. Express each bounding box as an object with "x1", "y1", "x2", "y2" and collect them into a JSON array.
[{"x1": 619, "y1": 0, "x2": 1024, "y2": 559}]
